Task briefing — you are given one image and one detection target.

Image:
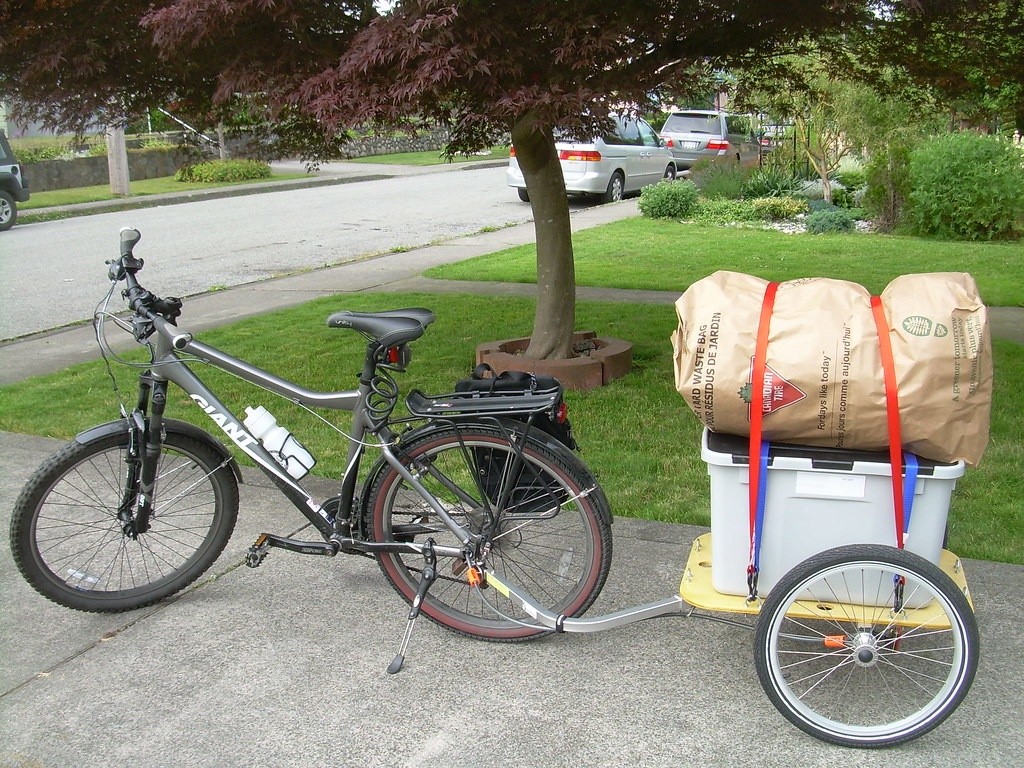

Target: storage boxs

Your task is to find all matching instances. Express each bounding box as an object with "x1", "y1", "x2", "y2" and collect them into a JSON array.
[{"x1": 696, "y1": 427, "x2": 967, "y2": 609}]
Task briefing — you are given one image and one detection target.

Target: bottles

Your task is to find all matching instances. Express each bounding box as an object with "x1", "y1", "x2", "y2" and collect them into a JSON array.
[{"x1": 242, "y1": 404, "x2": 316, "y2": 480}]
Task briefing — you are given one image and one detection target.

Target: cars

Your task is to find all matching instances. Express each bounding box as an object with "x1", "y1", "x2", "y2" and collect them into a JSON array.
[
  {"x1": 760, "y1": 131, "x2": 777, "y2": 155},
  {"x1": 509, "y1": 112, "x2": 677, "y2": 203}
]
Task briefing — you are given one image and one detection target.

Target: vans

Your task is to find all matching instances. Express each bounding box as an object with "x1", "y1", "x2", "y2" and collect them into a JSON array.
[{"x1": 659, "y1": 110, "x2": 760, "y2": 171}]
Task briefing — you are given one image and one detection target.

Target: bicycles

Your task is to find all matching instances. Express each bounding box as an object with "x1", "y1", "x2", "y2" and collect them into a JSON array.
[{"x1": 10, "y1": 226, "x2": 616, "y2": 674}]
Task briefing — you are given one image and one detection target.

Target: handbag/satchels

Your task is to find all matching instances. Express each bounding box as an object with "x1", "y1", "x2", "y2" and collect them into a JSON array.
[{"x1": 455, "y1": 363, "x2": 572, "y2": 514}]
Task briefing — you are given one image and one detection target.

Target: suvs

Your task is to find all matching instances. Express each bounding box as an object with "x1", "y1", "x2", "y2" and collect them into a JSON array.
[{"x1": 0, "y1": 129, "x2": 30, "y2": 231}]
